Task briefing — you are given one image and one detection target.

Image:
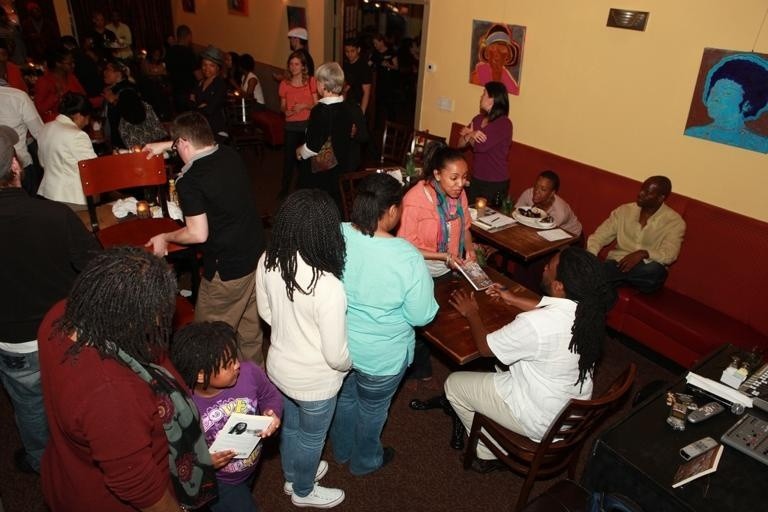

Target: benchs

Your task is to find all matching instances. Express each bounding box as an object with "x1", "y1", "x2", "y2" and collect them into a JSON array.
[{"x1": 447, "y1": 121, "x2": 768, "y2": 379}]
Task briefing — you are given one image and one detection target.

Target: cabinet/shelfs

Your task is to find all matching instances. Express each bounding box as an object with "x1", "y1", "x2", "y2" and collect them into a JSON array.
[{"x1": 582, "y1": 344, "x2": 768, "y2": 512}]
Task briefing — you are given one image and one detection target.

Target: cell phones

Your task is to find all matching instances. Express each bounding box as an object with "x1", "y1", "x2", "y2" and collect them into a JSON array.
[{"x1": 666, "y1": 403, "x2": 688, "y2": 432}]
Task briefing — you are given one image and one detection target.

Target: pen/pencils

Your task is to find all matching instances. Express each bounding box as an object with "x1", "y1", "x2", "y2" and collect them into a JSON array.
[
  {"x1": 500, "y1": 288, "x2": 508, "y2": 291},
  {"x1": 491, "y1": 217, "x2": 499, "y2": 222}
]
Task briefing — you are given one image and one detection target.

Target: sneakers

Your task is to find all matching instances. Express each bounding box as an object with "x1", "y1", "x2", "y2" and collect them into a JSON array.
[
  {"x1": 383, "y1": 447, "x2": 395, "y2": 462},
  {"x1": 283, "y1": 460, "x2": 345, "y2": 508}
]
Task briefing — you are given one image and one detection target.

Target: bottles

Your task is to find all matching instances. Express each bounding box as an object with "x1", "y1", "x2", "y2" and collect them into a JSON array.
[
  {"x1": 729, "y1": 356, "x2": 750, "y2": 375},
  {"x1": 406, "y1": 153, "x2": 415, "y2": 175},
  {"x1": 169, "y1": 178, "x2": 179, "y2": 206}
]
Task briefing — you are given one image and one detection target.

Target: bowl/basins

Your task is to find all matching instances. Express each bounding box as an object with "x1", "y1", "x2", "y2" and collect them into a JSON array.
[{"x1": 516, "y1": 207, "x2": 548, "y2": 222}]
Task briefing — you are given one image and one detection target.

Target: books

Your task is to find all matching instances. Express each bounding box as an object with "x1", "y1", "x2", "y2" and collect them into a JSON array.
[
  {"x1": 672, "y1": 444, "x2": 724, "y2": 489},
  {"x1": 720, "y1": 414, "x2": 768, "y2": 466}
]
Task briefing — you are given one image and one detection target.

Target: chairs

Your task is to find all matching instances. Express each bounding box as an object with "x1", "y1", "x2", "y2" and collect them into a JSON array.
[
  {"x1": 462, "y1": 363, "x2": 639, "y2": 510},
  {"x1": 76, "y1": 102, "x2": 447, "y2": 304}
]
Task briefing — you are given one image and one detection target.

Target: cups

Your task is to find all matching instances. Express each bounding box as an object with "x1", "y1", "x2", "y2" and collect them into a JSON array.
[
  {"x1": 476, "y1": 197, "x2": 487, "y2": 218},
  {"x1": 135, "y1": 200, "x2": 149, "y2": 219}
]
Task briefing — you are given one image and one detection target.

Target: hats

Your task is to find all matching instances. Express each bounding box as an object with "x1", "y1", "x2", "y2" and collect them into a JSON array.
[
  {"x1": 288, "y1": 27, "x2": 307, "y2": 40},
  {"x1": 199, "y1": 45, "x2": 224, "y2": 65}
]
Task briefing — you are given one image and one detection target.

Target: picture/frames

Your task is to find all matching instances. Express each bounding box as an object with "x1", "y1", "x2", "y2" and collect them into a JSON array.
[{"x1": 226, "y1": 0, "x2": 249, "y2": 18}]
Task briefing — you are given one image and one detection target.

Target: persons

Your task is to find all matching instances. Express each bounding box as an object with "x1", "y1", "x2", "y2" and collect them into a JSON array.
[
  {"x1": 444, "y1": 246, "x2": 622, "y2": 473},
  {"x1": 255, "y1": 143, "x2": 478, "y2": 509},
  {"x1": 274, "y1": 28, "x2": 420, "y2": 202},
  {"x1": 0, "y1": 10, "x2": 266, "y2": 212},
  {"x1": 514, "y1": 171, "x2": 582, "y2": 238},
  {"x1": 685, "y1": 53, "x2": 768, "y2": 155},
  {"x1": 36, "y1": 246, "x2": 283, "y2": 511},
  {"x1": 142, "y1": 114, "x2": 266, "y2": 370},
  {"x1": 456, "y1": 82, "x2": 513, "y2": 210},
  {"x1": 587, "y1": 176, "x2": 687, "y2": 294},
  {"x1": 1, "y1": 126, "x2": 104, "y2": 472}
]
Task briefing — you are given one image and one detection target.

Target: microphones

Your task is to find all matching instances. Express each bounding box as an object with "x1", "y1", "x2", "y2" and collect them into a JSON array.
[{"x1": 684, "y1": 383, "x2": 743, "y2": 416}]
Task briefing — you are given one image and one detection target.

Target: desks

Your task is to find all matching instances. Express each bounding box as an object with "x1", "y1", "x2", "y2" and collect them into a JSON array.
[
  {"x1": 410, "y1": 264, "x2": 542, "y2": 449},
  {"x1": 357, "y1": 165, "x2": 572, "y2": 287}
]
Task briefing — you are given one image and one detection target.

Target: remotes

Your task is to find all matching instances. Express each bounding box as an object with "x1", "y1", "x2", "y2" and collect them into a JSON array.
[
  {"x1": 679, "y1": 436, "x2": 718, "y2": 461},
  {"x1": 687, "y1": 402, "x2": 724, "y2": 423}
]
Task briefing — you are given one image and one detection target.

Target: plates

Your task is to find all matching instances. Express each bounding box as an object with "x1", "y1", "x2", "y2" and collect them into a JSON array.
[{"x1": 512, "y1": 212, "x2": 557, "y2": 229}]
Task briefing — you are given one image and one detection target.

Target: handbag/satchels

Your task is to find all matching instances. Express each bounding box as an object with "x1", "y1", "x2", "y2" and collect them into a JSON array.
[
  {"x1": 378, "y1": 54, "x2": 403, "y2": 85},
  {"x1": 310, "y1": 136, "x2": 339, "y2": 173},
  {"x1": 117, "y1": 101, "x2": 169, "y2": 148}
]
